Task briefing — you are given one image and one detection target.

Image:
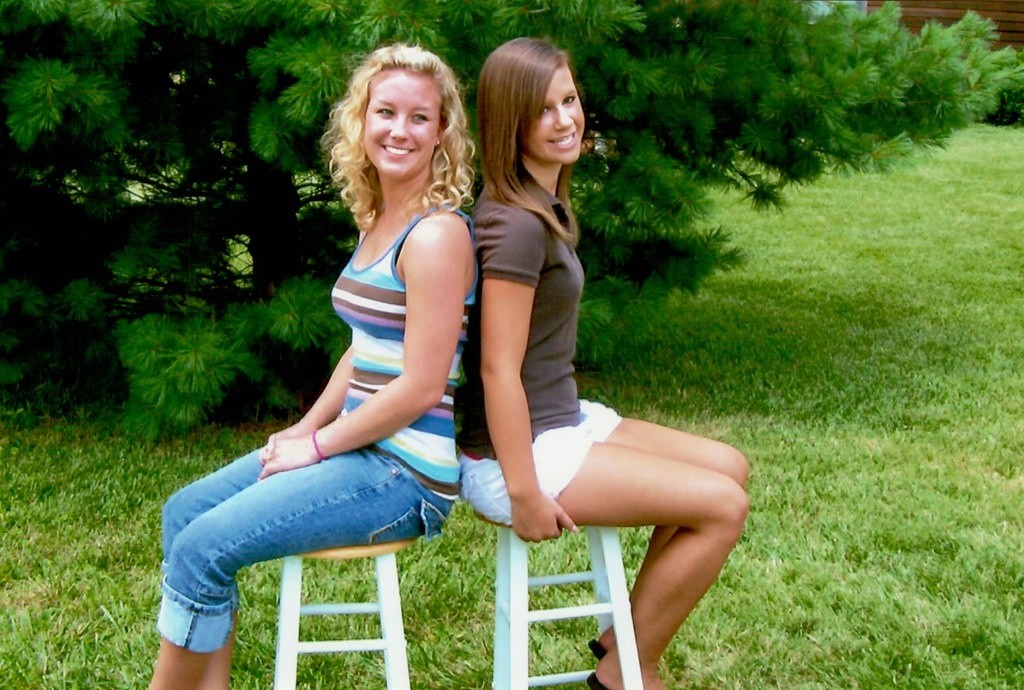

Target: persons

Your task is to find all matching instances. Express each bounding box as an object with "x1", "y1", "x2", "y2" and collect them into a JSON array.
[
  {"x1": 139, "y1": 41, "x2": 482, "y2": 690},
  {"x1": 450, "y1": 34, "x2": 750, "y2": 690}
]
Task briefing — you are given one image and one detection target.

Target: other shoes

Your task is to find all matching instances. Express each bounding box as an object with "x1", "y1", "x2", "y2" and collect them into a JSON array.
[
  {"x1": 589, "y1": 639, "x2": 606, "y2": 661},
  {"x1": 586, "y1": 672, "x2": 609, "y2": 690}
]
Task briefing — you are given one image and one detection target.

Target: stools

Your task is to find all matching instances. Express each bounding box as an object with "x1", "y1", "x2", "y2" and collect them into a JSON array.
[
  {"x1": 274, "y1": 536, "x2": 420, "y2": 690},
  {"x1": 473, "y1": 508, "x2": 644, "y2": 690}
]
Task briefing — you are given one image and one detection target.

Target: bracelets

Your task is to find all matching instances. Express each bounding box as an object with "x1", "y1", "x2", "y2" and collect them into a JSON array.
[{"x1": 311, "y1": 429, "x2": 327, "y2": 460}]
